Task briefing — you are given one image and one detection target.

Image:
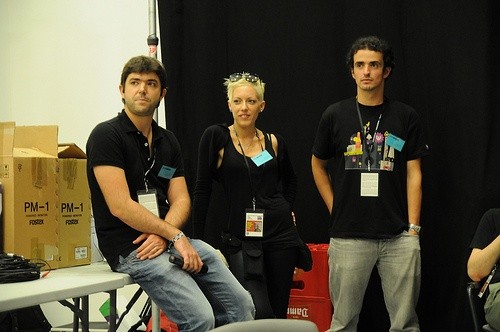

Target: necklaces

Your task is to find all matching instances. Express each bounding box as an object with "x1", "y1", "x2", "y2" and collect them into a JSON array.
[{"x1": 146, "y1": 127, "x2": 151, "y2": 139}]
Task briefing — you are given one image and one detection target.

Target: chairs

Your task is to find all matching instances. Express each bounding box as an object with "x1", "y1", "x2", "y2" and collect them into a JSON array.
[{"x1": 466, "y1": 282, "x2": 496, "y2": 332}]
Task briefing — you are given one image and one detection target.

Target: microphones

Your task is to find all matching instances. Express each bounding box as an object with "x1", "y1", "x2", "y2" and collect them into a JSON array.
[{"x1": 169, "y1": 254, "x2": 208, "y2": 274}]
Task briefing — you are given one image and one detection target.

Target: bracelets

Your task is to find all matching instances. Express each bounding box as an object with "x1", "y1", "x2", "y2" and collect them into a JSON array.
[
  {"x1": 407, "y1": 223, "x2": 421, "y2": 233},
  {"x1": 167, "y1": 231, "x2": 184, "y2": 249}
]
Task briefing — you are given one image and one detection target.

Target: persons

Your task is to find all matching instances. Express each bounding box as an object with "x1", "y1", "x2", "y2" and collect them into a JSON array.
[
  {"x1": 312, "y1": 35, "x2": 422, "y2": 332},
  {"x1": 86, "y1": 56, "x2": 256, "y2": 332},
  {"x1": 467, "y1": 209, "x2": 500, "y2": 332},
  {"x1": 191, "y1": 72, "x2": 303, "y2": 320}
]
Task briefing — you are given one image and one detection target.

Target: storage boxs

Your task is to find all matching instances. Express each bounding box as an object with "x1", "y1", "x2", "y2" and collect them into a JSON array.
[
  {"x1": 288, "y1": 295, "x2": 333, "y2": 332},
  {"x1": 0, "y1": 120, "x2": 60, "y2": 271},
  {"x1": 287, "y1": 241, "x2": 333, "y2": 298},
  {"x1": 58, "y1": 142, "x2": 92, "y2": 269}
]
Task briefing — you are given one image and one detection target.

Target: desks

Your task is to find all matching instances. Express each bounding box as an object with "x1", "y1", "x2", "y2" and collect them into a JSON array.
[{"x1": 0, "y1": 260, "x2": 162, "y2": 332}]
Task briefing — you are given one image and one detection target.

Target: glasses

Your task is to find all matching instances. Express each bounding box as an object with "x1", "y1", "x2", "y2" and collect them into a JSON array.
[{"x1": 229, "y1": 72, "x2": 261, "y2": 85}]
